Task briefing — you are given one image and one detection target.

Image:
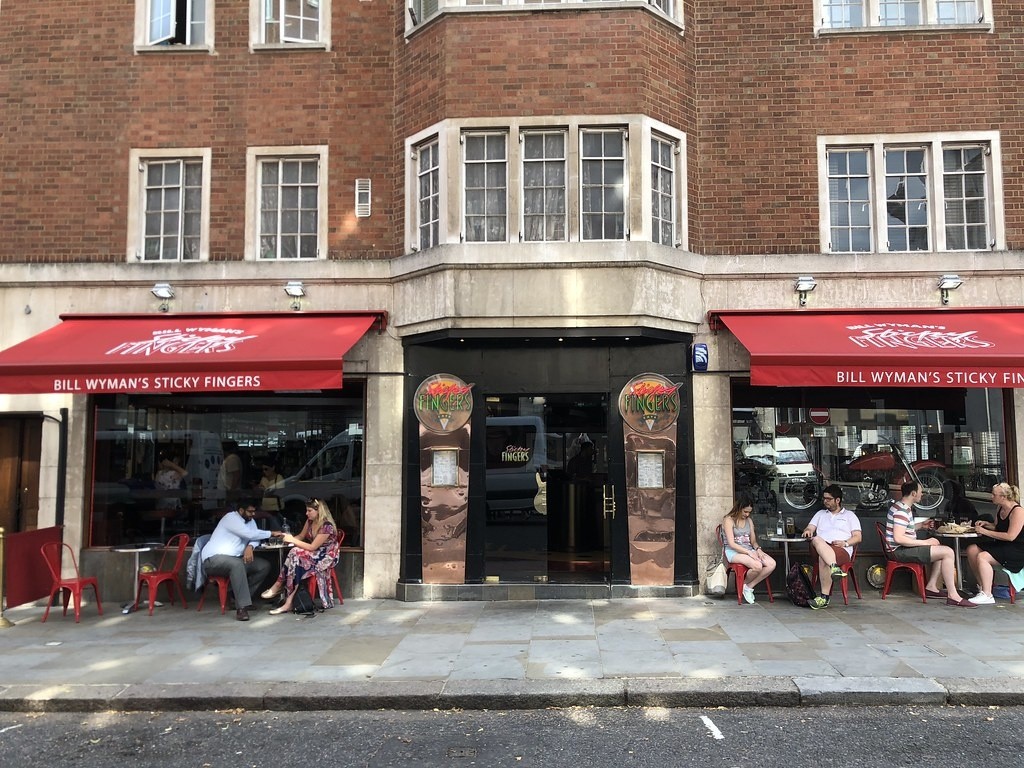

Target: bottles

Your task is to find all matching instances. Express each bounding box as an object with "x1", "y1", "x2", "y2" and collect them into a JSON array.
[
  {"x1": 281, "y1": 517, "x2": 290, "y2": 545},
  {"x1": 776, "y1": 511, "x2": 785, "y2": 537}
]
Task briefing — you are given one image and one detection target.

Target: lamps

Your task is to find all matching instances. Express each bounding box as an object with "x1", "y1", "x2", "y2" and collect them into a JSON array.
[
  {"x1": 283, "y1": 281, "x2": 307, "y2": 310},
  {"x1": 794, "y1": 276, "x2": 817, "y2": 305},
  {"x1": 934, "y1": 274, "x2": 965, "y2": 305},
  {"x1": 150, "y1": 282, "x2": 174, "y2": 312}
]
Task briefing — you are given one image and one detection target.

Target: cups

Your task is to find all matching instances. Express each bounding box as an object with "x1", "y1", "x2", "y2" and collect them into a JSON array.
[
  {"x1": 767, "y1": 528, "x2": 774, "y2": 537},
  {"x1": 269, "y1": 537, "x2": 284, "y2": 545},
  {"x1": 934, "y1": 518, "x2": 942, "y2": 529},
  {"x1": 960, "y1": 517, "x2": 968, "y2": 525}
]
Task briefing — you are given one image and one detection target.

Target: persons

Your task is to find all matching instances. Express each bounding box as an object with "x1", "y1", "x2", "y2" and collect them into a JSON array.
[
  {"x1": 885, "y1": 481, "x2": 976, "y2": 608},
  {"x1": 722, "y1": 497, "x2": 776, "y2": 604},
  {"x1": 801, "y1": 485, "x2": 862, "y2": 609},
  {"x1": 261, "y1": 498, "x2": 340, "y2": 614},
  {"x1": 256, "y1": 459, "x2": 285, "y2": 512},
  {"x1": 155, "y1": 455, "x2": 189, "y2": 528},
  {"x1": 202, "y1": 498, "x2": 286, "y2": 620},
  {"x1": 219, "y1": 439, "x2": 242, "y2": 505},
  {"x1": 966, "y1": 482, "x2": 1024, "y2": 604}
]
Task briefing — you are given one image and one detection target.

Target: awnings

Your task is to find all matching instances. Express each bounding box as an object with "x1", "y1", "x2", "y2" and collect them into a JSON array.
[
  {"x1": 719, "y1": 310, "x2": 1024, "y2": 388},
  {"x1": 0, "y1": 317, "x2": 378, "y2": 394}
]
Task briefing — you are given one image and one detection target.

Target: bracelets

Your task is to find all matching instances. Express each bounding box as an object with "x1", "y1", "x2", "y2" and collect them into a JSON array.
[{"x1": 756, "y1": 547, "x2": 762, "y2": 551}]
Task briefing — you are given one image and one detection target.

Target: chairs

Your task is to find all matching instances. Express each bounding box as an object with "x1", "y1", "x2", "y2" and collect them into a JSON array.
[
  {"x1": 135, "y1": 532, "x2": 190, "y2": 617},
  {"x1": 991, "y1": 566, "x2": 1024, "y2": 604},
  {"x1": 716, "y1": 524, "x2": 775, "y2": 604},
  {"x1": 309, "y1": 529, "x2": 345, "y2": 606},
  {"x1": 186, "y1": 533, "x2": 232, "y2": 615},
  {"x1": 874, "y1": 520, "x2": 928, "y2": 604},
  {"x1": 41, "y1": 539, "x2": 104, "y2": 625}
]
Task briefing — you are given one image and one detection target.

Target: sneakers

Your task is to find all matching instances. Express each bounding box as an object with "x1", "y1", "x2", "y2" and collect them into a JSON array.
[
  {"x1": 807, "y1": 594, "x2": 829, "y2": 610},
  {"x1": 968, "y1": 591, "x2": 996, "y2": 605},
  {"x1": 830, "y1": 564, "x2": 847, "y2": 582},
  {"x1": 743, "y1": 583, "x2": 755, "y2": 604}
]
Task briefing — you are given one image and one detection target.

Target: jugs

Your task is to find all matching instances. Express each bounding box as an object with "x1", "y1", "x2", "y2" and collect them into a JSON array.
[{"x1": 786, "y1": 517, "x2": 795, "y2": 538}]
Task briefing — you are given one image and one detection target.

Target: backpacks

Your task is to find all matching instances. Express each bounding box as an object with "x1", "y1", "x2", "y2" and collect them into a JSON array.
[{"x1": 786, "y1": 562, "x2": 816, "y2": 606}]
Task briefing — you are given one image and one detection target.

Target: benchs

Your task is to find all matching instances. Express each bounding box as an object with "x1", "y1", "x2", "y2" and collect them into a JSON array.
[{"x1": 812, "y1": 543, "x2": 859, "y2": 606}]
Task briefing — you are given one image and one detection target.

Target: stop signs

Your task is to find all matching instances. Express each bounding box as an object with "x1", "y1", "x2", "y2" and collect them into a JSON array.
[{"x1": 809, "y1": 408, "x2": 830, "y2": 425}]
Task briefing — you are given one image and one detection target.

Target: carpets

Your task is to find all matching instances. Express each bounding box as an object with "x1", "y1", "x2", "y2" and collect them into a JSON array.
[{"x1": 486, "y1": 554, "x2": 609, "y2": 573}]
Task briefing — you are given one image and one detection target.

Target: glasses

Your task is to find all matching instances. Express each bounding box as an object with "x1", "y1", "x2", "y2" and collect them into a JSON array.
[
  {"x1": 261, "y1": 468, "x2": 271, "y2": 472},
  {"x1": 245, "y1": 510, "x2": 254, "y2": 513},
  {"x1": 821, "y1": 496, "x2": 835, "y2": 501}
]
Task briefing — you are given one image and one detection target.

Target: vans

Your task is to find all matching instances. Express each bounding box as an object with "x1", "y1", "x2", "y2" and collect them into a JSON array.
[
  {"x1": 260, "y1": 416, "x2": 549, "y2": 530},
  {"x1": 773, "y1": 437, "x2": 816, "y2": 492},
  {"x1": 92, "y1": 429, "x2": 227, "y2": 530}
]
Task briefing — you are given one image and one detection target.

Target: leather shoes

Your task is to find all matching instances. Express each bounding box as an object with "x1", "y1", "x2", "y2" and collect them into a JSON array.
[
  {"x1": 246, "y1": 605, "x2": 256, "y2": 610},
  {"x1": 237, "y1": 607, "x2": 249, "y2": 620}
]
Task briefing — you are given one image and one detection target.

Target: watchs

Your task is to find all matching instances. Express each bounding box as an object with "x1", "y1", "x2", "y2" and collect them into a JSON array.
[{"x1": 844, "y1": 540, "x2": 848, "y2": 547}]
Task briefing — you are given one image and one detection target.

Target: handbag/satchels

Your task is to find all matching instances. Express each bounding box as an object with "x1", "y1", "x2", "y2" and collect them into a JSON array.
[
  {"x1": 708, "y1": 564, "x2": 728, "y2": 596},
  {"x1": 294, "y1": 590, "x2": 317, "y2": 615}
]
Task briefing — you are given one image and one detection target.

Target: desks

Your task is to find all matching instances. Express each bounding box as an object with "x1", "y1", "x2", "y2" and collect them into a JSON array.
[
  {"x1": 758, "y1": 532, "x2": 806, "y2": 605},
  {"x1": 254, "y1": 543, "x2": 296, "y2": 612},
  {"x1": 926, "y1": 530, "x2": 983, "y2": 595},
  {"x1": 108, "y1": 542, "x2": 166, "y2": 613}
]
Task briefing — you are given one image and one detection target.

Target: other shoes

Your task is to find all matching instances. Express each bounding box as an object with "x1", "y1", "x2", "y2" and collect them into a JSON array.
[
  {"x1": 270, "y1": 604, "x2": 294, "y2": 615},
  {"x1": 924, "y1": 588, "x2": 948, "y2": 599},
  {"x1": 261, "y1": 589, "x2": 282, "y2": 599},
  {"x1": 946, "y1": 595, "x2": 978, "y2": 608}
]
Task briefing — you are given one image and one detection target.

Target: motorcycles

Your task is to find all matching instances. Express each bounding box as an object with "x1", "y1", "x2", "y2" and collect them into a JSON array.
[{"x1": 783, "y1": 435, "x2": 945, "y2": 511}]
